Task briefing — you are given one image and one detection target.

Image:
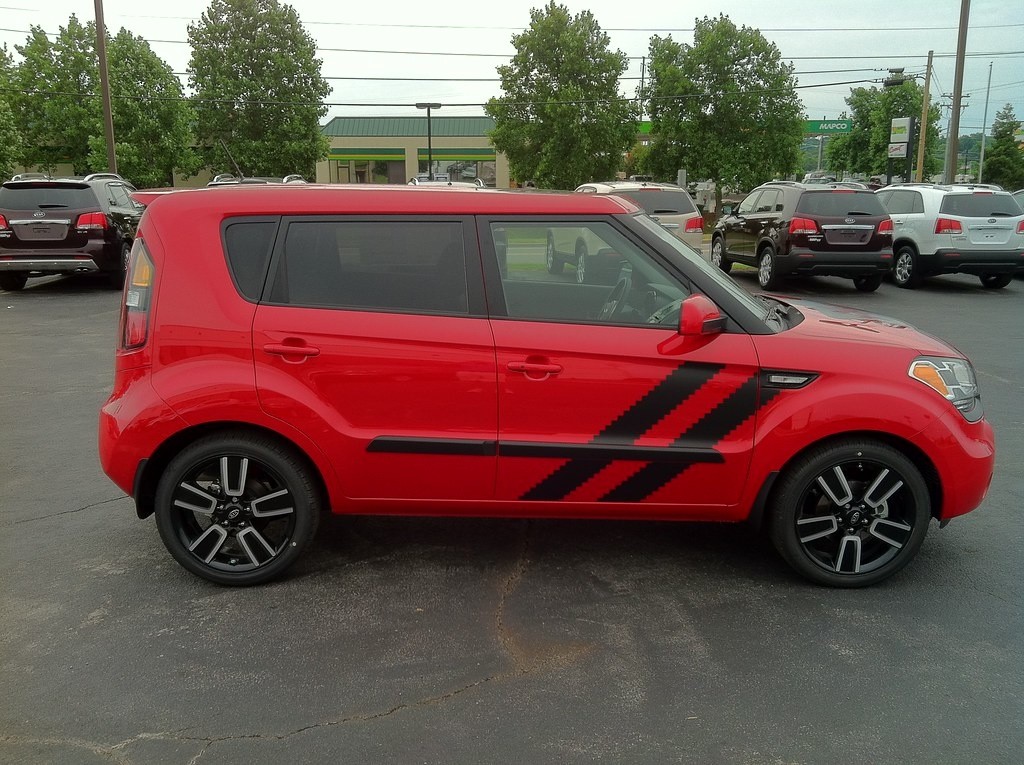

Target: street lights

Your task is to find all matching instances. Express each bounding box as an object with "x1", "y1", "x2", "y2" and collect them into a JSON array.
[{"x1": 416, "y1": 102, "x2": 441, "y2": 179}]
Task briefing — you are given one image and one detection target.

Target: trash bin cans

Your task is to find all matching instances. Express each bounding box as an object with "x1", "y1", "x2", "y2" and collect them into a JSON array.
[
  {"x1": 696, "y1": 204, "x2": 706, "y2": 213},
  {"x1": 721, "y1": 200, "x2": 738, "y2": 215}
]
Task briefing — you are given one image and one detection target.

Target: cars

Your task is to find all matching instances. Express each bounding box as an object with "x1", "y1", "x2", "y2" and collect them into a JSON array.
[{"x1": 92, "y1": 180, "x2": 998, "y2": 589}]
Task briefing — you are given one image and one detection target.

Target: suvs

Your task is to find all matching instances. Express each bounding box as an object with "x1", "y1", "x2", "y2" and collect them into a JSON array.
[
  {"x1": 871, "y1": 179, "x2": 1024, "y2": 292},
  {"x1": 208, "y1": 173, "x2": 508, "y2": 281},
  {"x1": 0, "y1": 171, "x2": 148, "y2": 291},
  {"x1": 711, "y1": 179, "x2": 896, "y2": 296},
  {"x1": 545, "y1": 179, "x2": 704, "y2": 284}
]
